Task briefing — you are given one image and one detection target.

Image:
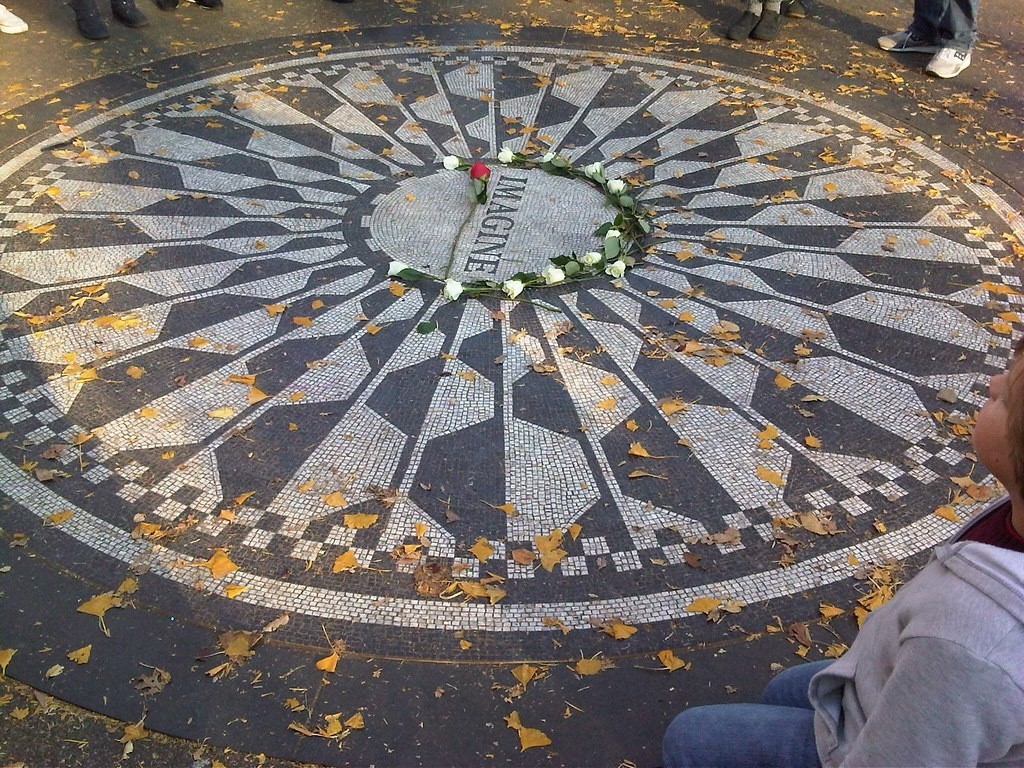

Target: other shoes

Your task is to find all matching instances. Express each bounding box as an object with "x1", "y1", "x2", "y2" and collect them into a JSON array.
[
  {"x1": 752, "y1": 3, "x2": 784, "y2": 40},
  {"x1": 157, "y1": 0, "x2": 179, "y2": 11},
  {"x1": 729, "y1": 10, "x2": 762, "y2": 41},
  {"x1": 185, "y1": 0, "x2": 223, "y2": 9}
]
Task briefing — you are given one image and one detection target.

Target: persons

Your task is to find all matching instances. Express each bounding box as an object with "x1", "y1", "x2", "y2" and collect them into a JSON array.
[
  {"x1": 785, "y1": 0, "x2": 812, "y2": 18},
  {"x1": 68, "y1": 0, "x2": 150, "y2": 39},
  {"x1": 661, "y1": 333, "x2": 1024, "y2": 768},
  {"x1": 0, "y1": 4, "x2": 28, "y2": 35},
  {"x1": 155, "y1": 0, "x2": 223, "y2": 11},
  {"x1": 728, "y1": 0, "x2": 782, "y2": 40},
  {"x1": 876, "y1": 0, "x2": 979, "y2": 78}
]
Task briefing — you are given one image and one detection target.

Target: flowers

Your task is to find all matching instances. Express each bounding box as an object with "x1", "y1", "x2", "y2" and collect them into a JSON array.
[{"x1": 385, "y1": 136, "x2": 665, "y2": 313}]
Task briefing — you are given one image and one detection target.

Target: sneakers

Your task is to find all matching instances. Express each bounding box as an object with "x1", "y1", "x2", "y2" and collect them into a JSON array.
[
  {"x1": 877, "y1": 30, "x2": 942, "y2": 53},
  {"x1": 69, "y1": 0, "x2": 110, "y2": 39},
  {"x1": 111, "y1": 0, "x2": 147, "y2": 27},
  {"x1": 925, "y1": 46, "x2": 972, "y2": 79},
  {"x1": 0, "y1": 4, "x2": 28, "y2": 34},
  {"x1": 786, "y1": 0, "x2": 812, "y2": 18}
]
